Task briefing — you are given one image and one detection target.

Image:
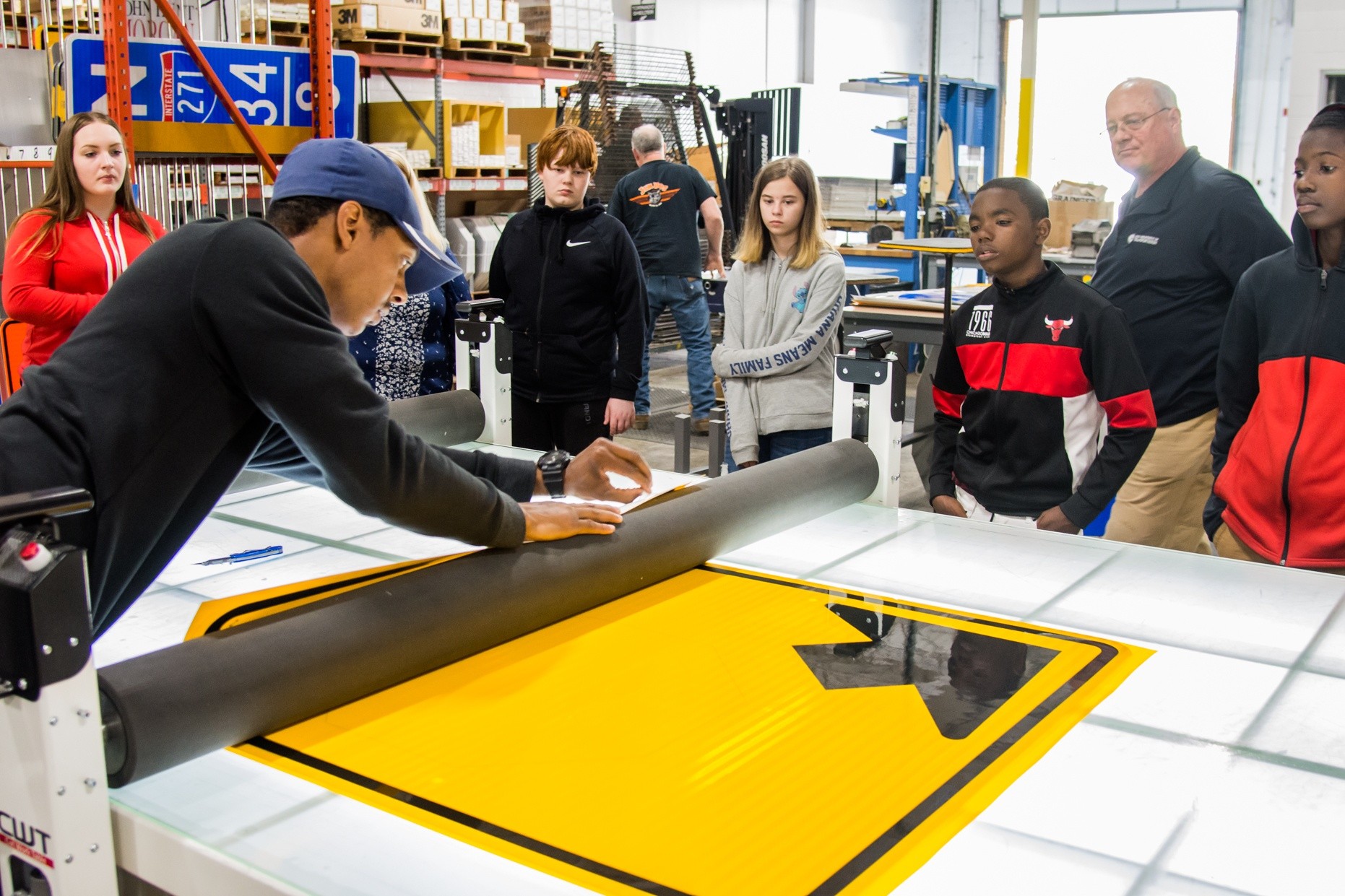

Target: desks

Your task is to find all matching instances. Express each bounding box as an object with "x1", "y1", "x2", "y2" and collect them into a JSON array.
[
  {"x1": 700, "y1": 266, "x2": 902, "y2": 285},
  {"x1": 841, "y1": 283, "x2": 990, "y2": 424},
  {"x1": 89, "y1": 440, "x2": 1344, "y2": 896},
  {"x1": 830, "y1": 243, "x2": 916, "y2": 282},
  {"x1": 824, "y1": 210, "x2": 905, "y2": 232},
  {"x1": 1045, "y1": 255, "x2": 1096, "y2": 283}
]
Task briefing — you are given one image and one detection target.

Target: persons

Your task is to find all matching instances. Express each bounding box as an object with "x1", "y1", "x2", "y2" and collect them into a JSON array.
[
  {"x1": 1203, "y1": 105, "x2": 1345, "y2": 576},
  {"x1": 1, "y1": 112, "x2": 169, "y2": 398},
  {"x1": 607, "y1": 124, "x2": 726, "y2": 434},
  {"x1": 490, "y1": 125, "x2": 652, "y2": 458},
  {"x1": 928, "y1": 177, "x2": 1156, "y2": 536},
  {"x1": 1090, "y1": 78, "x2": 1292, "y2": 561},
  {"x1": 1, "y1": 139, "x2": 654, "y2": 703},
  {"x1": 712, "y1": 156, "x2": 846, "y2": 473}
]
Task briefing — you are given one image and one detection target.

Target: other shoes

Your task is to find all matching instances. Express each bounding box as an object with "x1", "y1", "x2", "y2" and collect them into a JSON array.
[
  {"x1": 690, "y1": 419, "x2": 710, "y2": 436},
  {"x1": 633, "y1": 416, "x2": 649, "y2": 430}
]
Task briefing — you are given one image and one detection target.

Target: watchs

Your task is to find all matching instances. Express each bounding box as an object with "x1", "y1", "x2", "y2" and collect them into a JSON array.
[{"x1": 536, "y1": 446, "x2": 572, "y2": 498}]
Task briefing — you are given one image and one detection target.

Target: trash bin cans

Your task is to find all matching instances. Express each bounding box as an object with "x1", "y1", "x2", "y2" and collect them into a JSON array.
[{"x1": 868, "y1": 281, "x2": 915, "y2": 293}]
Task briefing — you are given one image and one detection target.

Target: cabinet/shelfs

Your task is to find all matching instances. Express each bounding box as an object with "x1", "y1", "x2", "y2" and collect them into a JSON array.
[{"x1": 360, "y1": 51, "x2": 616, "y2": 299}]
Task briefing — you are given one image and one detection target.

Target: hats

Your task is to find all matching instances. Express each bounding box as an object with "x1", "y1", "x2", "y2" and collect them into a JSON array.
[{"x1": 271, "y1": 138, "x2": 462, "y2": 295}]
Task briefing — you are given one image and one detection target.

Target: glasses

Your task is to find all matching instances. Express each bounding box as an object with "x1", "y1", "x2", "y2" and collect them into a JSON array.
[{"x1": 1098, "y1": 107, "x2": 1171, "y2": 141}]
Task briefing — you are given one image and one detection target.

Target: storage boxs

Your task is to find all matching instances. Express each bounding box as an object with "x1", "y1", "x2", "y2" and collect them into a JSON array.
[
  {"x1": 239, "y1": 0, "x2": 615, "y2": 52},
  {"x1": 1045, "y1": 200, "x2": 1116, "y2": 251}
]
